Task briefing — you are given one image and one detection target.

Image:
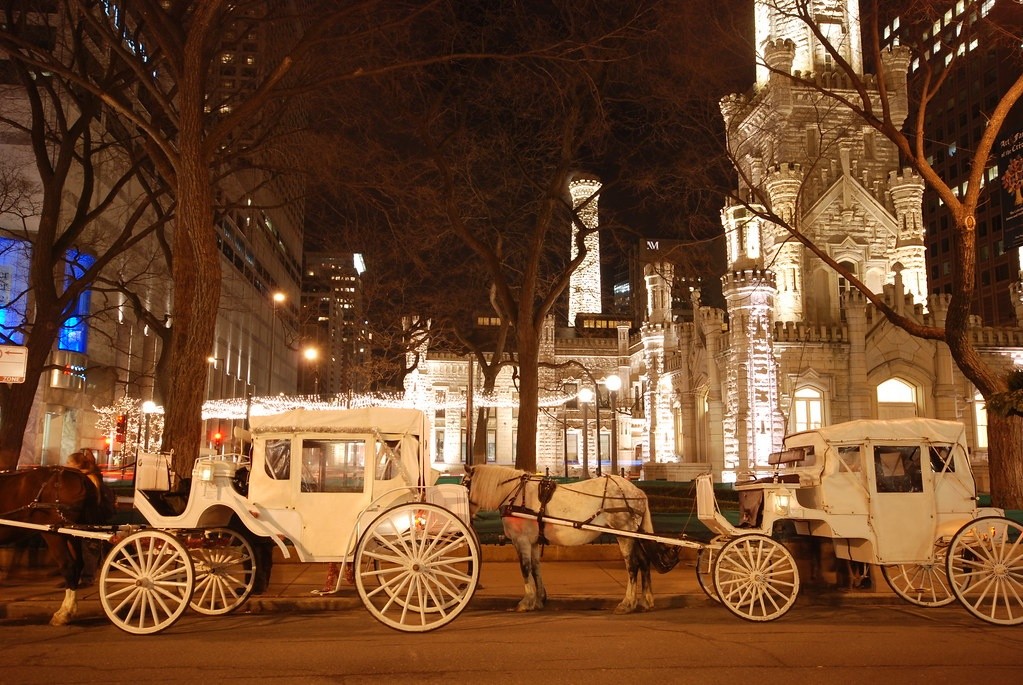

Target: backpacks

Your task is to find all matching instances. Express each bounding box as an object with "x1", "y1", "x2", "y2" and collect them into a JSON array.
[{"x1": 98, "y1": 485, "x2": 117, "y2": 522}]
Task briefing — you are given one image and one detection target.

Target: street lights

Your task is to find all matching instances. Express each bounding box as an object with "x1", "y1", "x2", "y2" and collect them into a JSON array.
[
  {"x1": 579, "y1": 387, "x2": 593, "y2": 479},
  {"x1": 267, "y1": 293, "x2": 285, "y2": 395},
  {"x1": 605, "y1": 376, "x2": 622, "y2": 473},
  {"x1": 142, "y1": 401, "x2": 155, "y2": 453},
  {"x1": 208, "y1": 357, "x2": 224, "y2": 453},
  {"x1": 301, "y1": 348, "x2": 316, "y2": 393}
]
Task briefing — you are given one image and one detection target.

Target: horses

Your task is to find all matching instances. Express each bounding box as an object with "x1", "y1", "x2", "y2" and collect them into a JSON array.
[
  {"x1": 0, "y1": 466, "x2": 99, "y2": 626},
  {"x1": 460, "y1": 464, "x2": 680, "y2": 615}
]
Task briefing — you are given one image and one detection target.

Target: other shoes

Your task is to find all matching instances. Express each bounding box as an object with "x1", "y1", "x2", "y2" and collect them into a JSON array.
[{"x1": 235, "y1": 586, "x2": 262, "y2": 595}]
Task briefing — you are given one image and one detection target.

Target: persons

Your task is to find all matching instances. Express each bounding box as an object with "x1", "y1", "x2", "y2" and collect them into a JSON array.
[{"x1": 67, "y1": 448, "x2": 104, "y2": 585}]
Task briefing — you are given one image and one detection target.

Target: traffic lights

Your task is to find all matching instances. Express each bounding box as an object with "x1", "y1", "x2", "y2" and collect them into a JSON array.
[
  {"x1": 117, "y1": 414, "x2": 124, "y2": 433},
  {"x1": 105, "y1": 438, "x2": 110, "y2": 449},
  {"x1": 214, "y1": 434, "x2": 221, "y2": 450},
  {"x1": 116, "y1": 435, "x2": 125, "y2": 442}
]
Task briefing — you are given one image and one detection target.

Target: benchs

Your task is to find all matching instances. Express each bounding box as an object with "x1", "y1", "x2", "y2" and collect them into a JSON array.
[
  {"x1": 177, "y1": 452, "x2": 235, "y2": 495},
  {"x1": 734, "y1": 470, "x2": 799, "y2": 493}
]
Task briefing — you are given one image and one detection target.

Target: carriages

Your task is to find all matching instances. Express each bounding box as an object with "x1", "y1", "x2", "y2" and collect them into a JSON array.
[
  {"x1": 458, "y1": 415, "x2": 1023, "y2": 626},
  {"x1": 0, "y1": 406, "x2": 484, "y2": 637}
]
